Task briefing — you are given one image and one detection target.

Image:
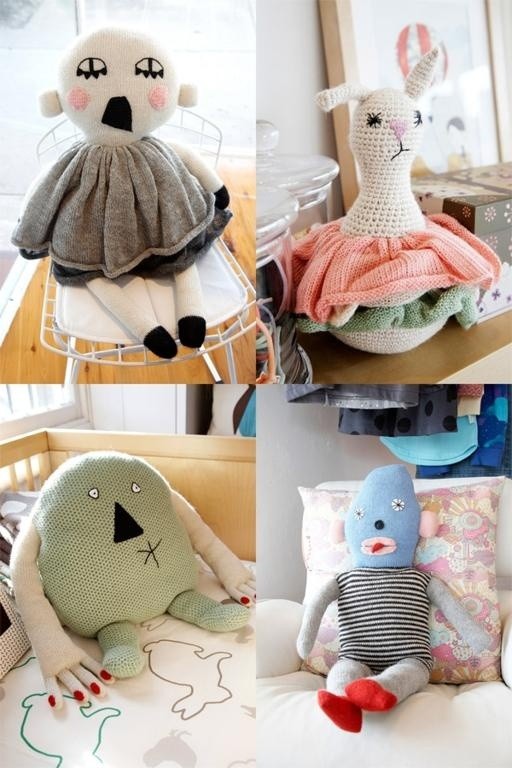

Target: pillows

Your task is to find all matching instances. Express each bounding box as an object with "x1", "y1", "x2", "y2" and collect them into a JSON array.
[{"x1": 296, "y1": 474, "x2": 504, "y2": 687}]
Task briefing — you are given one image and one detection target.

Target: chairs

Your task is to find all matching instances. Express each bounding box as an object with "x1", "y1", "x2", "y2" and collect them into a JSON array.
[
  {"x1": 30, "y1": 103, "x2": 257, "y2": 385},
  {"x1": 257, "y1": 466, "x2": 512, "y2": 768}
]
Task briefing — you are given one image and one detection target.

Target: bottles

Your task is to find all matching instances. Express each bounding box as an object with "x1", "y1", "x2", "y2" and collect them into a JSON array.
[{"x1": 256, "y1": 119, "x2": 340, "y2": 383}]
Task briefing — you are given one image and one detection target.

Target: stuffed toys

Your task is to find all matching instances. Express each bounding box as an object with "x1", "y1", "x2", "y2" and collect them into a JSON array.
[
  {"x1": 288, "y1": 44, "x2": 502, "y2": 355},
  {"x1": 296, "y1": 465, "x2": 489, "y2": 733},
  {"x1": 10, "y1": 450, "x2": 256, "y2": 714},
  {"x1": 12, "y1": 23, "x2": 233, "y2": 361}
]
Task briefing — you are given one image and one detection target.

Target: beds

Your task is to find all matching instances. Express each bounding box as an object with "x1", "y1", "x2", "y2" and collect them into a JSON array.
[{"x1": 0, "y1": 427, "x2": 256, "y2": 768}]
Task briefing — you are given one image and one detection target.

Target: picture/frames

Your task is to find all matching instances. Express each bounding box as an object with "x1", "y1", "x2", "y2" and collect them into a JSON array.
[{"x1": 318, "y1": 0, "x2": 512, "y2": 219}]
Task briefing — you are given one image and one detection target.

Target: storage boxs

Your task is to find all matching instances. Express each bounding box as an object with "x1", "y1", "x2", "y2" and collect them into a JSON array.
[{"x1": 401, "y1": 158, "x2": 511, "y2": 327}]
